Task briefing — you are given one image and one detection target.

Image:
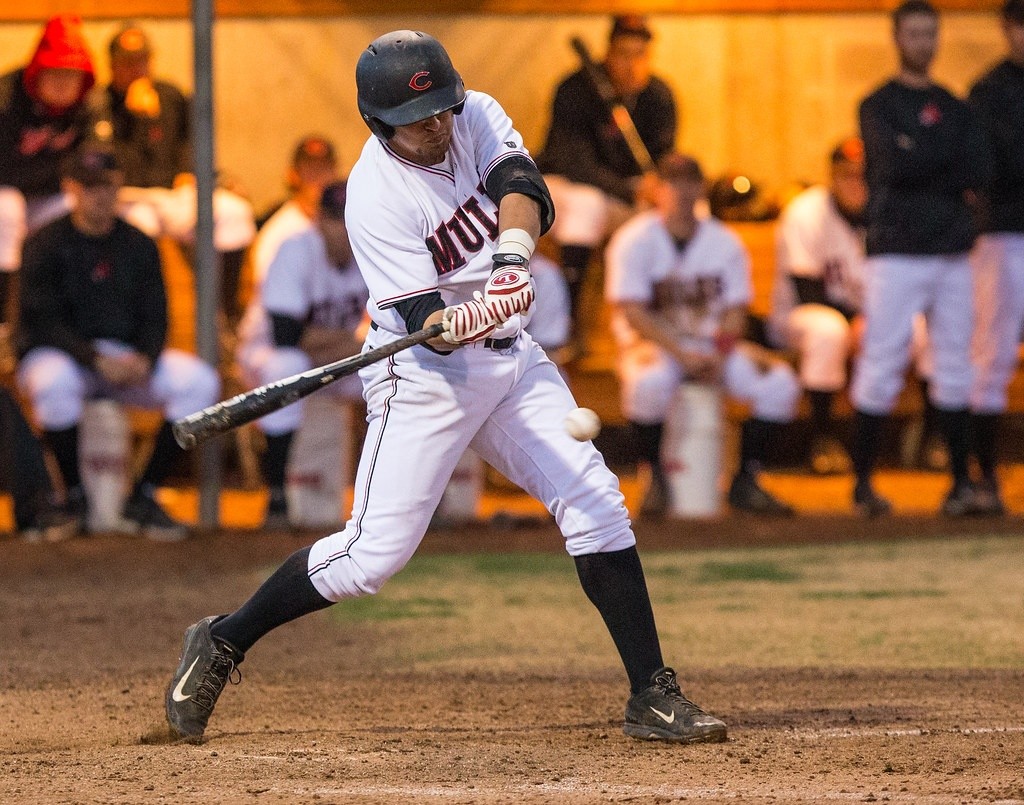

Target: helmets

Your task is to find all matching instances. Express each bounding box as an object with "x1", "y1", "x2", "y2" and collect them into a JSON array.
[{"x1": 356, "y1": 29, "x2": 467, "y2": 145}]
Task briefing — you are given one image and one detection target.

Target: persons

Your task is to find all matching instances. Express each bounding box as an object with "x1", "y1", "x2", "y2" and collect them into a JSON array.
[
  {"x1": 769, "y1": 139, "x2": 951, "y2": 472},
  {"x1": 533, "y1": 15, "x2": 679, "y2": 362},
  {"x1": 0, "y1": 387, "x2": 54, "y2": 543},
  {"x1": 233, "y1": 135, "x2": 371, "y2": 534},
  {"x1": 0, "y1": 10, "x2": 97, "y2": 323},
  {"x1": 847, "y1": 0, "x2": 1024, "y2": 524},
  {"x1": 82, "y1": 26, "x2": 254, "y2": 322},
  {"x1": 603, "y1": 154, "x2": 797, "y2": 519},
  {"x1": 516, "y1": 248, "x2": 570, "y2": 348},
  {"x1": 164, "y1": 30, "x2": 731, "y2": 745},
  {"x1": 12, "y1": 142, "x2": 219, "y2": 542}
]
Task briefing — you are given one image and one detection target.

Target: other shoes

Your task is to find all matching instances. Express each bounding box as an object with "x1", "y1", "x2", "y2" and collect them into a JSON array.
[
  {"x1": 115, "y1": 492, "x2": 187, "y2": 543},
  {"x1": 729, "y1": 474, "x2": 794, "y2": 517},
  {"x1": 936, "y1": 482, "x2": 978, "y2": 516},
  {"x1": 261, "y1": 491, "x2": 292, "y2": 534},
  {"x1": 967, "y1": 483, "x2": 1004, "y2": 519},
  {"x1": 26, "y1": 505, "x2": 86, "y2": 543},
  {"x1": 853, "y1": 486, "x2": 892, "y2": 518},
  {"x1": 641, "y1": 475, "x2": 668, "y2": 516},
  {"x1": 810, "y1": 429, "x2": 851, "y2": 475}
]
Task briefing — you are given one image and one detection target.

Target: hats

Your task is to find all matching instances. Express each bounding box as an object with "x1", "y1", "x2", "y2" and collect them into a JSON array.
[
  {"x1": 609, "y1": 14, "x2": 653, "y2": 42},
  {"x1": 294, "y1": 134, "x2": 335, "y2": 169},
  {"x1": 321, "y1": 180, "x2": 348, "y2": 217},
  {"x1": 110, "y1": 30, "x2": 151, "y2": 62},
  {"x1": 67, "y1": 149, "x2": 119, "y2": 188},
  {"x1": 653, "y1": 154, "x2": 704, "y2": 184},
  {"x1": 832, "y1": 135, "x2": 869, "y2": 166}
]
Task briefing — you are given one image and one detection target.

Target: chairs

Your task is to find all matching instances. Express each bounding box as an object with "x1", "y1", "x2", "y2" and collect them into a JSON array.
[{"x1": 0, "y1": 170, "x2": 1024, "y2": 543}]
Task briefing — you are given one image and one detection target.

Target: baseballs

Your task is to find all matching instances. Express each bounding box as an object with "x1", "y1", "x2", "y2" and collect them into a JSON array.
[{"x1": 564, "y1": 406, "x2": 602, "y2": 442}]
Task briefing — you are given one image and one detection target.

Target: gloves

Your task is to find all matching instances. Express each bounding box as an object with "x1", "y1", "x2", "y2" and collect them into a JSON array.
[
  {"x1": 440, "y1": 291, "x2": 493, "y2": 344},
  {"x1": 483, "y1": 253, "x2": 536, "y2": 329}
]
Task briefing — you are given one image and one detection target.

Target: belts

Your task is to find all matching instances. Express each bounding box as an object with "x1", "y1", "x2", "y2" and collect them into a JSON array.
[{"x1": 370, "y1": 320, "x2": 518, "y2": 350}]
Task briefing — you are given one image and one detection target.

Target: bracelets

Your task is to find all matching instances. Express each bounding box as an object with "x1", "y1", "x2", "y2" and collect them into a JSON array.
[{"x1": 497, "y1": 227, "x2": 536, "y2": 262}]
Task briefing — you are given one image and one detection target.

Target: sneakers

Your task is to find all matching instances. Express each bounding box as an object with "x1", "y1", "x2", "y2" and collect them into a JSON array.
[
  {"x1": 621, "y1": 667, "x2": 728, "y2": 744},
  {"x1": 164, "y1": 613, "x2": 246, "y2": 743}
]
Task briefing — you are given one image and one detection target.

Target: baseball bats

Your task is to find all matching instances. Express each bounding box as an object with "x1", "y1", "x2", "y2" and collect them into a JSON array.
[
  {"x1": 568, "y1": 33, "x2": 659, "y2": 182},
  {"x1": 171, "y1": 285, "x2": 537, "y2": 452}
]
